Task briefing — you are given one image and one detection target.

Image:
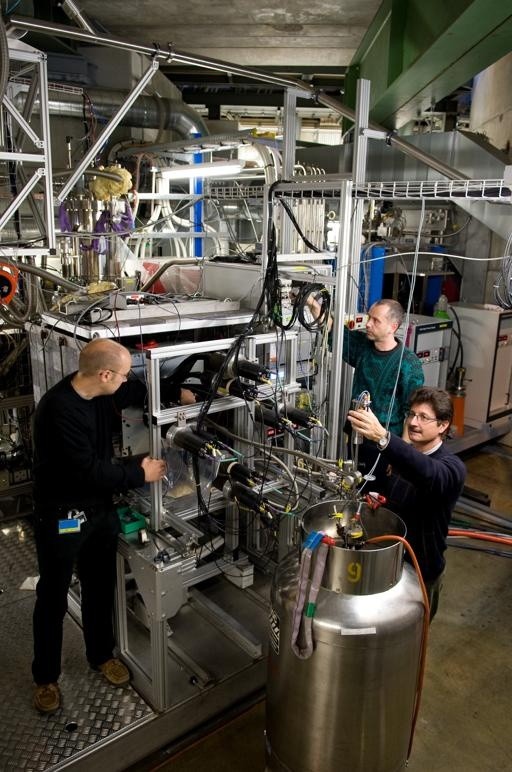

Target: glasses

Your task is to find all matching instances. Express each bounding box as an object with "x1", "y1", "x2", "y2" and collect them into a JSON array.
[{"x1": 404, "y1": 411, "x2": 439, "y2": 421}]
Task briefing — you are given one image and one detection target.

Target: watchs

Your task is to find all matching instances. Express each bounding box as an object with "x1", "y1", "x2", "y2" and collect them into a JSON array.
[{"x1": 379, "y1": 432, "x2": 389, "y2": 449}]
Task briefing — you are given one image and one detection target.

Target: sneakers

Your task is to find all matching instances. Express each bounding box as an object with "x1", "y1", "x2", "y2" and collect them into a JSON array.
[
  {"x1": 34, "y1": 684, "x2": 60, "y2": 713},
  {"x1": 99, "y1": 658, "x2": 130, "y2": 684}
]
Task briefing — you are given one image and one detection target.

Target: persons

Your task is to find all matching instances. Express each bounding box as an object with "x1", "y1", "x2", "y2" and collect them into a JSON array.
[
  {"x1": 32, "y1": 338, "x2": 197, "y2": 713},
  {"x1": 349, "y1": 389, "x2": 466, "y2": 619},
  {"x1": 306, "y1": 294, "x2": 424, "y2": 476}
]
